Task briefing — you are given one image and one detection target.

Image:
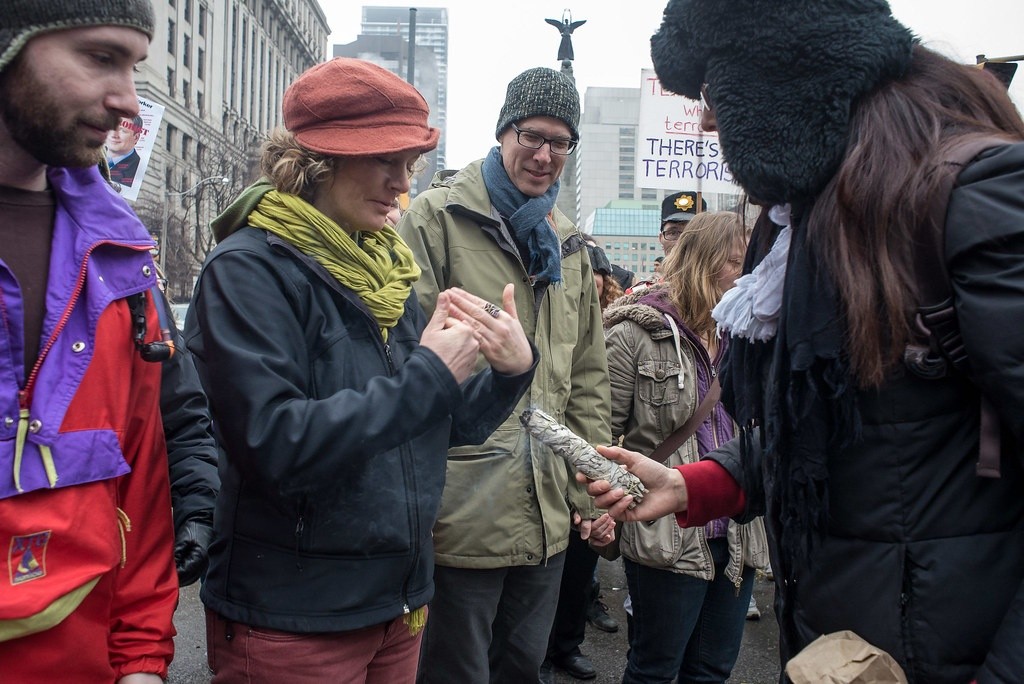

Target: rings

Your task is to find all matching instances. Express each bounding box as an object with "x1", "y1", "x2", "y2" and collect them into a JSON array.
[{"x1": 483, "y1": 302, "x2": 502, "y2": 318}]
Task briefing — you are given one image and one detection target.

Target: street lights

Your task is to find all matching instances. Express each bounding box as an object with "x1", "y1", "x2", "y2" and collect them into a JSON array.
[{"x1": 159, "y1": 175, "x2": 229, "y2": 273}]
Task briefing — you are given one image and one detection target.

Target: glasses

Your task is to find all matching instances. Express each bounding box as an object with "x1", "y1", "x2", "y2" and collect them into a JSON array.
[
  {"x1": 511, "y1": 121, "x2": 579, "y2": 155},
  {"x1": 694, "y1": 83, "x2": 712, "y2": 114},
  {"x1": 661, "y1": 229, "x2": 683, "y2": 241}
]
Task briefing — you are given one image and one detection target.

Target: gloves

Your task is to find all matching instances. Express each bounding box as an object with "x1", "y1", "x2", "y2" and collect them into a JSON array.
[{"x1": 177, "y1": 510, "x2": 211, "y2": 585}]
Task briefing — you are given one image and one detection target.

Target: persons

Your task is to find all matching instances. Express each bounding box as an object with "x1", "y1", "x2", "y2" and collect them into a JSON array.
[
  {"x1": 385, "y1": 197, "x2": 405, "y2": 229},
  {"x1": 104, "y1": 115, "x2": 143, "y2": 188},
  {"x1": 541, "y1": 192, "x2": 773, "y2": 684},
  {"x1": 153, "y1": 262, "x2": 220, "y2": 587},
  {"x1": 183, "y1": 57, "x2": 541, "y2": 684},
  {"x1": 557, "y1": 9, "x2": 574, "y2": 60},
  {"x1": 0, "y1": 0, "x2": 179, "y2": 684},
  {"x1": 393, "y1": 67, "x2": 612, "y2": 684},
  {"x1": 576, "y1": 0, "x2": 1024, "y2": 684}
]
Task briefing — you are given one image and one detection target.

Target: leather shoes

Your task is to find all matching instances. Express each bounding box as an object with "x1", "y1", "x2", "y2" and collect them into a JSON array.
[
  {"x1": 564, "y1": 650, "x2": 598, "y2": 680},
  {"x1": 591, "y1": 600, "x2": 621, "y2": 633}
]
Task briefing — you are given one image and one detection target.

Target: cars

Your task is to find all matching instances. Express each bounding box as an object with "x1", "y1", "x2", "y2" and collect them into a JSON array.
[{"x1": 170, "y1": 302, "x2": 190, "y2": 332}]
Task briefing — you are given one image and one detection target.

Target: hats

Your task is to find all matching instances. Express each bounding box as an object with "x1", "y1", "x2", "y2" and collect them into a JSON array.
[
  {"x1": 650, "y1": 0, "x2": 920, "y2": 198},
  {"x1": 281, "y1": 54, "x2": 439, "y2": 156},
  {"x1": 495, "y1": 68, "x2": 582, "y2": 142},
  {"x1": 659, "y1": 192, "x2": 709, "y2": 224},
  {"x1": 0, "y1": 0, "x2": 155, "y2": 73}
]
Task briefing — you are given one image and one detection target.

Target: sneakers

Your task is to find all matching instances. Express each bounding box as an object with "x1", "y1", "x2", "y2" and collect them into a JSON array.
[{"x1": 747, "y1": 598, "x2": 761, "y2": 622}]
